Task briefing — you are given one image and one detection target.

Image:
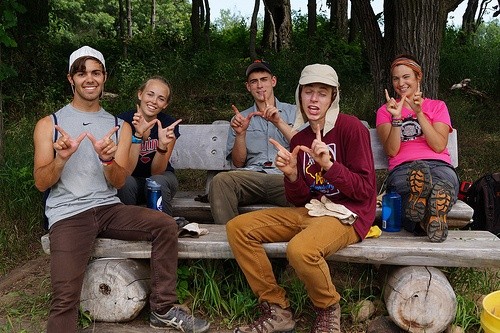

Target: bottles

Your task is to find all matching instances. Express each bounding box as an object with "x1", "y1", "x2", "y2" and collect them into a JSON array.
[
  {"x1": 382, "y1": 185, "x2": 402, "y2": 232},
  {"x1": 145, "y1": 177, "x2": 164, "y2": 212}
]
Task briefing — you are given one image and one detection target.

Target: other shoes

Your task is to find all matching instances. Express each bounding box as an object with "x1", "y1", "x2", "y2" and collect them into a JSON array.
[
  {"x1": 406, "y1": 159, "x2": 432, "y2": 223},
  {"x1": 423, "y1": 180, "x2": 457, "y2": 243}
]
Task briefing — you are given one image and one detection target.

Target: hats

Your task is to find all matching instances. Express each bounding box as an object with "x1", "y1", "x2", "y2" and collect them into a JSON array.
[
  {"x1": 69, "y1": 46, "x2": 106, "y2": 99},
  {"x1": 290, "y1": 64, "x2": 340, "y2": 137},
  {"x1": 246, "y1": 60, "x2": 271, "y2": 77}
]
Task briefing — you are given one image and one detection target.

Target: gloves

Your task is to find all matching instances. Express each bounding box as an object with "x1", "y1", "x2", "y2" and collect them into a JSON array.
[
  {"x1": 305, "y1": 195, "x2": 359, "y2": 226},
  {"x1": 178, "y1": 223, "x2": 208, "y2": 238},
  {"x1": 172, "y1": 217, "x2": 189, "y2": 230}
]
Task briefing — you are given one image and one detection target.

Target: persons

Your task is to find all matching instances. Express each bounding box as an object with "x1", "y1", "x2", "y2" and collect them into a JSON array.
[
  {"x1": 33, "y1": 45, "x2": 210, "y2": 333},
  {"x1": 207, "y1": 58, "x2": 301, "y2": 296},
  {"x1": 224, "y1": 64, "x2": 378, "y2": 332},
  {"x1": 375, "y1": 55, "x2": 459, "y2": 242}
]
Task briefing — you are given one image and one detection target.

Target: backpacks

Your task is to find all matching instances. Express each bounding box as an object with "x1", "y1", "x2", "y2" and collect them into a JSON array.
[{"x1": 462, "y1": 172, "x2": 500, "y2": 237}]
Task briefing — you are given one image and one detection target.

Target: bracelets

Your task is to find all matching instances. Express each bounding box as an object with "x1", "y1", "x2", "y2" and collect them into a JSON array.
[
  {"x1": 97, "y1": 157, "x2": 115, "y2": 165},
  {"x1": 156, "y1": 145, "x2": 167, "y2": 153},
  {"x1": 393, "y1": 119, "x2": 404, "y2": 127}
]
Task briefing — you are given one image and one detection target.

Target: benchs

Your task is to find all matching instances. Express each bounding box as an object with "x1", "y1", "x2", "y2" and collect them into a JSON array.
[
  {"x1": 41, "y1": 224, "x2": 500, "y2": 333},
  {"x1": 168, "y1": 121, "x2": 474, "y2": 227}
]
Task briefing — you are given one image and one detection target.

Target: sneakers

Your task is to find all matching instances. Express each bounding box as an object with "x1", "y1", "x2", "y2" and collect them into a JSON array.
[
  {"x1": 150, "y1": 306, "x2": 211, "y2": 333},
  {"x1": 233, "y1": 300, "x2": 297, "y2": 333},
  {"x1": 313, "y1": 301, "x2": 340, "y2": 333}
]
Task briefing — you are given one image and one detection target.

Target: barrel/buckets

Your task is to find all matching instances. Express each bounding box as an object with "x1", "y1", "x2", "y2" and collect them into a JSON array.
[{"x1": 480, "y1": 290, "x2": 500, "y2": 333}]
